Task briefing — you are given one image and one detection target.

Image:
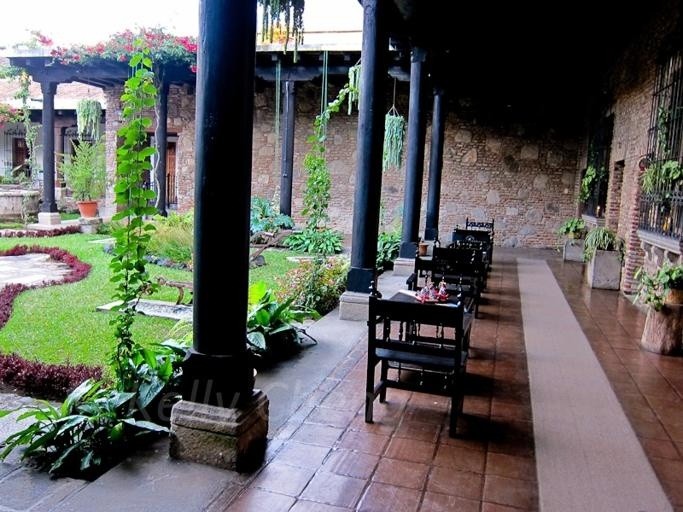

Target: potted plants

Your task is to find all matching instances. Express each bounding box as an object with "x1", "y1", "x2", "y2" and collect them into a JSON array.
[
  {"x1": 55, "y1": 135, "x2": 105, "y2": 218},
  {"x1": 554, "y1": 217, "x2": 584, "y2": 238},
  {"x1": 633, "y1": 263, "x2": 683, "y2": 310},
  {"x1": 582, "y1": 226, "x2": 626, "y2": 263}
]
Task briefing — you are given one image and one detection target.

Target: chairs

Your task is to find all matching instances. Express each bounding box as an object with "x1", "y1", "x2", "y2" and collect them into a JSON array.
[{"x1": 365, "y1": 216, "x2": 495, "y2": 438}]
